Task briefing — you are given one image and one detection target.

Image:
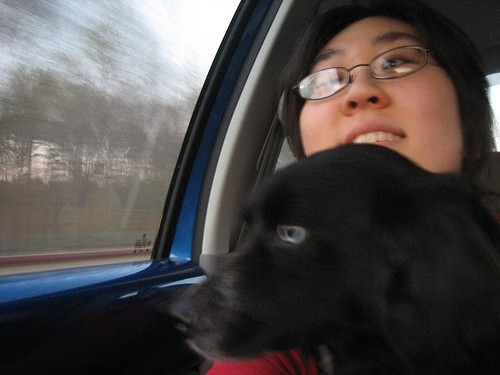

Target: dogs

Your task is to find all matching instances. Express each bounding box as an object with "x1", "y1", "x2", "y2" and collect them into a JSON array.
[{"x1": 168, "y1": 143, "x2": 500, "y2": 375}]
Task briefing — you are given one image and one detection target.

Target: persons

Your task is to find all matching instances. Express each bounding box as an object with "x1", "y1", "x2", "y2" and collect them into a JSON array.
[{"x1": 204, "y1": 0, "x2": 500, "y2": 375}]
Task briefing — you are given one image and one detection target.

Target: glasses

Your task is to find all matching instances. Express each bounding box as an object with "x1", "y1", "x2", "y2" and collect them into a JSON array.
[{"x1": 289, "y1": 45, "x2": 434, "y2": 101}]
